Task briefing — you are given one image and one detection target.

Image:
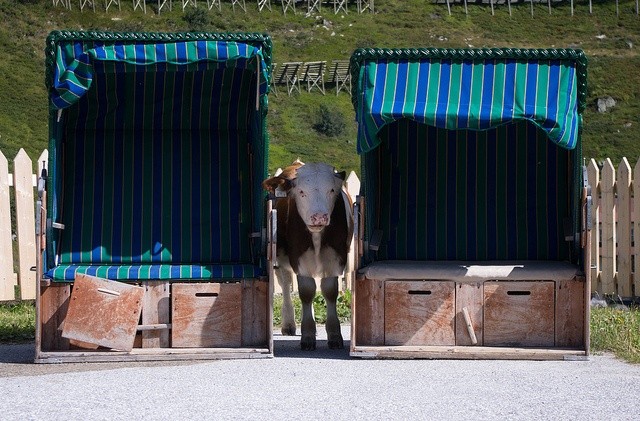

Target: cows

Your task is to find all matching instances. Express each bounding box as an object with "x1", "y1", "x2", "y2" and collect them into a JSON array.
[{"x1": 261, "y1": 158, "x2": 355, "y2": 352}]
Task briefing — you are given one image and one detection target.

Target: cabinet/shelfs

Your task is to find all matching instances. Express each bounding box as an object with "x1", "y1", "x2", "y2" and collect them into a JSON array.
[
  {"x1": 33, "y1": 278, "x2": 273, "y2": 364},
  {"x1": 349, "y1": 276, "x2": 591, "y2": 363}
]
